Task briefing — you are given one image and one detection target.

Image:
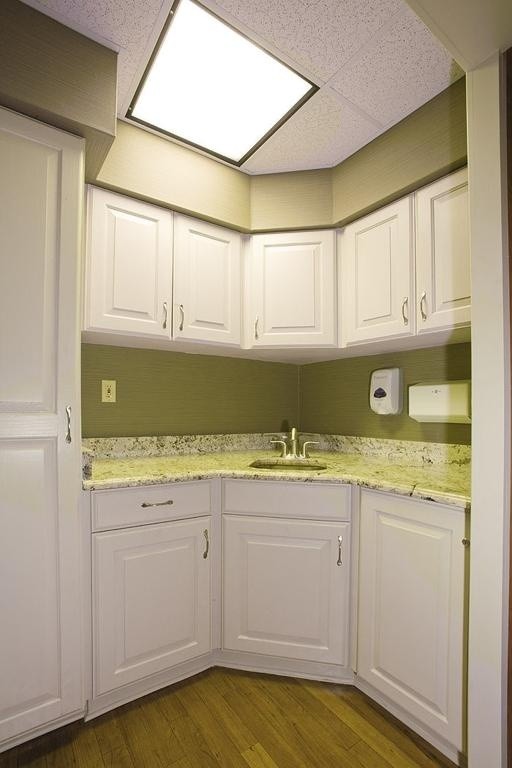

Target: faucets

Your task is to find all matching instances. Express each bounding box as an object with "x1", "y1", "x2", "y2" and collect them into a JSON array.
[{"x1": 291, "y1": 428, "x2": 299, "y2": 454}]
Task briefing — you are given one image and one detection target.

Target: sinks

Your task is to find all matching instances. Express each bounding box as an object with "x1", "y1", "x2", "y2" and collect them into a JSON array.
[{"x1": 248, "y1": 459, "x2": 328, "y2": 471}]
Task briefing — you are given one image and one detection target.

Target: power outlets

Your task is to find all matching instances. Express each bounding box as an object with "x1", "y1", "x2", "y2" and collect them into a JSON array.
[{"x1": 101, "y1": 380, "x2": 117, "y2": 402}]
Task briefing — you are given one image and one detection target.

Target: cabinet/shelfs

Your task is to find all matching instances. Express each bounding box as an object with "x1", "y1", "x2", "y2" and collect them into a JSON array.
[
  {"x1": 223, "y1": 479, "x2": 351, "y2": 669},
  {"x1": 341, "y1": 160, "x2": 471, "y2": 358},
  {"x1": 356, "y1": 487, "x2": 464, "y2": 756},
  {"x1": 84, "y1": 184, "x2": 245, "y2": 358},
  {"x1": 246, "y1": 229, "x2": 341, "y2": 366},
  {"x1": 1, "y1": 104, "x2": 84, "y2": 756},
  {"x1": 91, "y1": 480, "x2": 211, "y2": 698}
]
